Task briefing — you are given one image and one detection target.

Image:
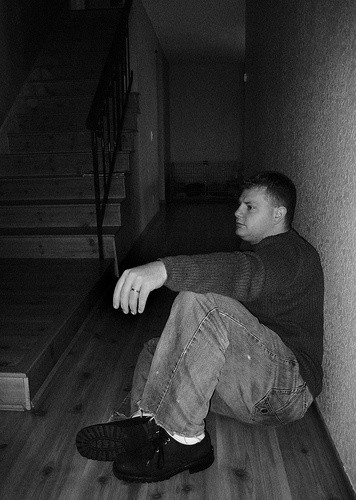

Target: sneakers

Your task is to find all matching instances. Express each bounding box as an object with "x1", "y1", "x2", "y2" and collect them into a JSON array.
[{"x1": 76, "y1": 415, "x2": 214, "y2": 484}]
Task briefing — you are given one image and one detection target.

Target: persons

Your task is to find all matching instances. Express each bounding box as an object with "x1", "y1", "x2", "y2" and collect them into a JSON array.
[{"x1": 76, "y1": 171, "x2": 324, "y2": 483}]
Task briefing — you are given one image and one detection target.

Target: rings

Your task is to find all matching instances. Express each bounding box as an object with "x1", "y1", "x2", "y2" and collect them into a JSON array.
[{"x1": 131, "y1": 288, "x2": 140, "y2": 293}]
